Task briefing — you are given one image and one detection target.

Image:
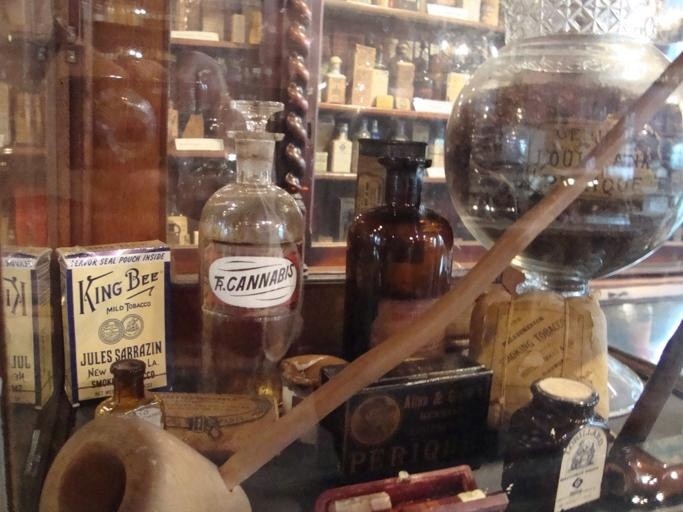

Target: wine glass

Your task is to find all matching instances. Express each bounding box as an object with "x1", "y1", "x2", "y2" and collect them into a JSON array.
[{"x1": 445, "y1": 31, "x2": 683, "y2": 419}]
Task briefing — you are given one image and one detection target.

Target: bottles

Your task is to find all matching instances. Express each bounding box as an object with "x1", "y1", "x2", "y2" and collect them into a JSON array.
[
  {"x1": 194, "y1": 99, "x2": 306, "y2": 415},
  {"x1": 500, "y1": 375, "x2": 616, "y2": 511},
  {"x1": 94, "y1": 358, "x2": 165, "y2": 438},
  {"x1": 341, "y1": 159, "x2": 454, "y2": 371},
  {"x1": 322, "y1": 35, "x2": 470, "y2": 180}
]
